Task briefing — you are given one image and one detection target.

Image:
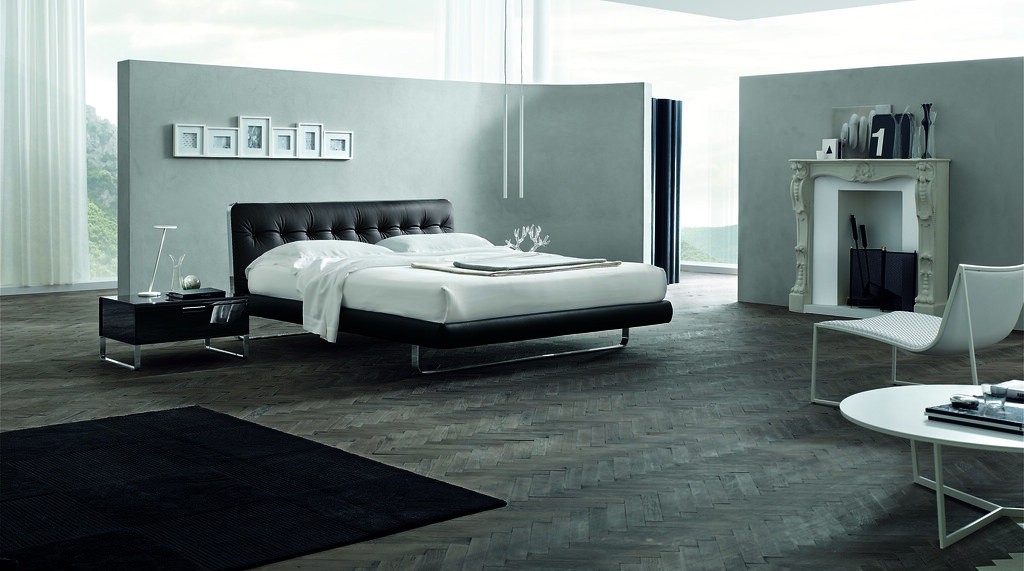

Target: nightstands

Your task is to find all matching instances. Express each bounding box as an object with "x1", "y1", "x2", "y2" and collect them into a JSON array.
[{"x1": 99, "y1": 292, "x2": 250, "y2": 371}]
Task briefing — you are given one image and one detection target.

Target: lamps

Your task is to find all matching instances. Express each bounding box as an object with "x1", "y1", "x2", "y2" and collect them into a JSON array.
[{"x1": 137, "y1": 224, "x2": 178, "y2": 297}]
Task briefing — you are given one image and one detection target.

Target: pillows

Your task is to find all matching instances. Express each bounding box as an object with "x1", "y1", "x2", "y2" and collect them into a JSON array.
[
  {"x1": 244, "y1": 240, "x2": 395, "y2": 280},
  {"x1": 374, "y1": 233, "x2": 494, "y2": 252}
]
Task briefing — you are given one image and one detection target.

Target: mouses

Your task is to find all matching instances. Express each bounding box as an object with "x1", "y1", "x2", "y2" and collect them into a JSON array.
[{"x1": 951, "y1": 395, "x2": 979, "y2": 409}]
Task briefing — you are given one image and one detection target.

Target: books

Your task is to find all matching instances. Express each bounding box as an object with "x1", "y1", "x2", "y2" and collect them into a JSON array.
[
  {"x1": 166, "y1": 287, "x2": 226, "y2": 298},
  {"x1": 924, "y1": 379, "x2": 1024, "y2": 435}
]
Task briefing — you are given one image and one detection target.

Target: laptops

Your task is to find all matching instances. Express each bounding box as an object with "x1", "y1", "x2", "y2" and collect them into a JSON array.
[{"x1": 925, "y1": 400, "x2": 1024, "y2": 434}]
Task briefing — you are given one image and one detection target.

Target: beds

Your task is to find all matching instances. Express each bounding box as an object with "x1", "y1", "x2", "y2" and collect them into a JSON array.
[{"x1": 227, "y1": 198, "x2": 673, "y2": 374}]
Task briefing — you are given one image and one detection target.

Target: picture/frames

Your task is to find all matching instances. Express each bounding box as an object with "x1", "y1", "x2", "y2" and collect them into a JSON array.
[{"x1": 173, "y1": 116, "x2": 353, "y2": 160}]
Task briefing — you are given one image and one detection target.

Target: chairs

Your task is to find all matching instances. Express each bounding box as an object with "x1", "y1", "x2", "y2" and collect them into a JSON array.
[{"x1": 810, "y1": 264, "x2": 1024, "y2": 406}]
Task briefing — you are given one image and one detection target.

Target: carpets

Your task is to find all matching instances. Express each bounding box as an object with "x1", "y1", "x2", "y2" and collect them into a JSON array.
[{"x1": 0, "y1": 406, "x2": 506, "y2": 571}]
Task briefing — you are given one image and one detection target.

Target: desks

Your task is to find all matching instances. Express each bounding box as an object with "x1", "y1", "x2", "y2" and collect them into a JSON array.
[{"x1": 839, "y1": 383, "x2": 1024, "y2": 548}]
